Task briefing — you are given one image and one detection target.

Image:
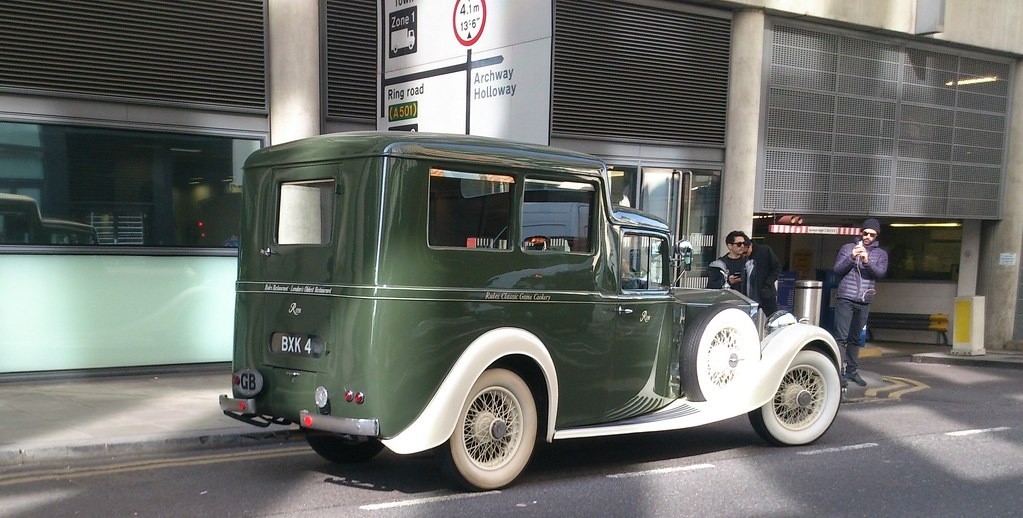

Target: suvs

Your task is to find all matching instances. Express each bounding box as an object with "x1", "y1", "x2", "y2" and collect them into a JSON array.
[{"x1": 219, "y1": 130, "x2": 842, "y2": 494}]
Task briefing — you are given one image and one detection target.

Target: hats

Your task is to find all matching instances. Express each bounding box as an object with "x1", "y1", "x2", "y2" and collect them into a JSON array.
[{"x1": 860, "y1": 219, "x2": 881, "y2": 234}]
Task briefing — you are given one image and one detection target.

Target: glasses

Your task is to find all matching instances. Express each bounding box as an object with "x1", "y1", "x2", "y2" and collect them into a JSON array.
[
  {"x1": 862, "y1": 231, "x2": 877, "y2": 237},
  {"x1": 733, "y1": 241, "x2": 749, "y2": 247},
  {"x1": 742, "y1": 245, "x2": 750, "y2": 254}
]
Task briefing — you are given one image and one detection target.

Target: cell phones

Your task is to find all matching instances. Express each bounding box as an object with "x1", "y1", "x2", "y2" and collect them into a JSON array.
[
  {"x1": 858, "y1": 240, "x2": 863, "y2": 246},
  {"x1": 734, "y1": 272, "x2": 741, "y2": 278}
]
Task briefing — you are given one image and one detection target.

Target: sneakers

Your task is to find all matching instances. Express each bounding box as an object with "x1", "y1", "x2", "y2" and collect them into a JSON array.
[
  {"x1": 838, "y1": 373, "x2": 848, "y2": 387},
  {"x1": 844, "y1": 369, "x2": 867, "y2": 386}
]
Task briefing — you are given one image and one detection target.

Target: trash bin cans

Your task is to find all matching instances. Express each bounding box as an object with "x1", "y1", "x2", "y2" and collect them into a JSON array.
[{"x1": 794, "y1": 280, "x2": 823, "y2": 326}]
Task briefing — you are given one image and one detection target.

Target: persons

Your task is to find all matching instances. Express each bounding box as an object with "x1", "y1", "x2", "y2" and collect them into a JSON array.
[
  {"x1": 826, "y1": 219, "x2": 889, "y2": 386},
  {"x1": 705, "y1": 230, "x2": 781, "y2": 317}
]
